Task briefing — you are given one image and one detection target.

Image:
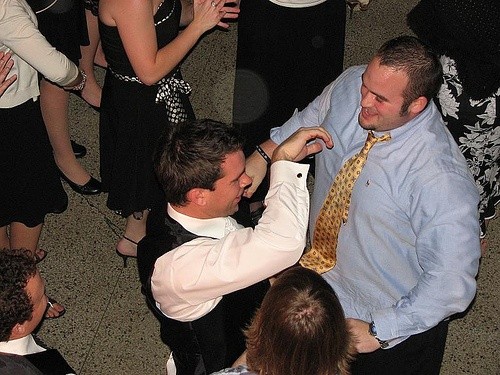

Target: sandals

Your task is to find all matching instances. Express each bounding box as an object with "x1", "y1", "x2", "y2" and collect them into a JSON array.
[
  {"x1": 34, "y1": 249, "x2": 47, "y2": 264},
  {"x1": 42, "y1": 298, "x2": 66, "y2": 320}
]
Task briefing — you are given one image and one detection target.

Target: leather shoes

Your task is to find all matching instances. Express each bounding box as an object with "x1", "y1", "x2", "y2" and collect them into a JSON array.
[
  {"x1": 70, "y1": 140, "x2": 87, "y2": 158},
  {"x1": 54, "y1": 160, "x2": 102, "y2": 195}
]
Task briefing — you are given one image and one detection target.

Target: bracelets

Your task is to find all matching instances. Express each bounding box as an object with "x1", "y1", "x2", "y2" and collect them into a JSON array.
[
  {"x1": 63, "y1": 68, "x2": 87, "y2": 90},
  {"x1": 255, "y1": 144, "x2": 273, "y2": 166}
]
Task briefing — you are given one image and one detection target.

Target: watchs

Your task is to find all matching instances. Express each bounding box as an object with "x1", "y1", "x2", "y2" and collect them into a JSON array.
[{"x1": 368, "y1": 320, "x2": 389, "y2": 349}]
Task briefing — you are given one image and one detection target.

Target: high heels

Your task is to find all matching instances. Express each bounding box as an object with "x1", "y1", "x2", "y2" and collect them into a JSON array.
[{"x1": 115, "y1": 235, "x2": 138, "y2": 268}]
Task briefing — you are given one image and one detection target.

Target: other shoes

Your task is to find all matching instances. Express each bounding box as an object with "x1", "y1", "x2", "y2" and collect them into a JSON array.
[
  {"x1": 70, "y1": 90, "x2": 100, "y2": 112},
  {"x1": 93, "y1": 63, "x2": 107, "y2": 70}
]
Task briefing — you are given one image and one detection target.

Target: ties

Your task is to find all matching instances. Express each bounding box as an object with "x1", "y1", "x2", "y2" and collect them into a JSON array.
[{"x1": 298, "y1": 133, "x2": 391, "y2": 275}]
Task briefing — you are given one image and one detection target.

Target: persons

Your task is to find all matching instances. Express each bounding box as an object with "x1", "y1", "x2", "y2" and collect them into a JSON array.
[
  {"x1": 239, "y1": 35, "x2": 482, "y2": 375},
  {"x1": 137, "y1": 119, "x2": 334, "y2": 375},
  {"x1": 0, "y1": 248, "x2": 79, "y2": 375},
  {"x1": 0, "y1": 0, "x2": 107, "y2": 263},
  {"x1": 98, "y1": 0, "x2": 240, "y2": 268},
  {"x1": 233, "y1": 0, "x2": 370, "y2": 230},
  {"x1": 207, "y1": 265, "x2": 359, "y2": 375},
  {"x1": 406, "y1": 0, "x2": 500, "y2": 239}
]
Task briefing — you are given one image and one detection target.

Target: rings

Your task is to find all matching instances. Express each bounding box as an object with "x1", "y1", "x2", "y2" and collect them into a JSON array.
[{"x1": 211, "y1": 1, "x2": 216, "y2": 7}]
